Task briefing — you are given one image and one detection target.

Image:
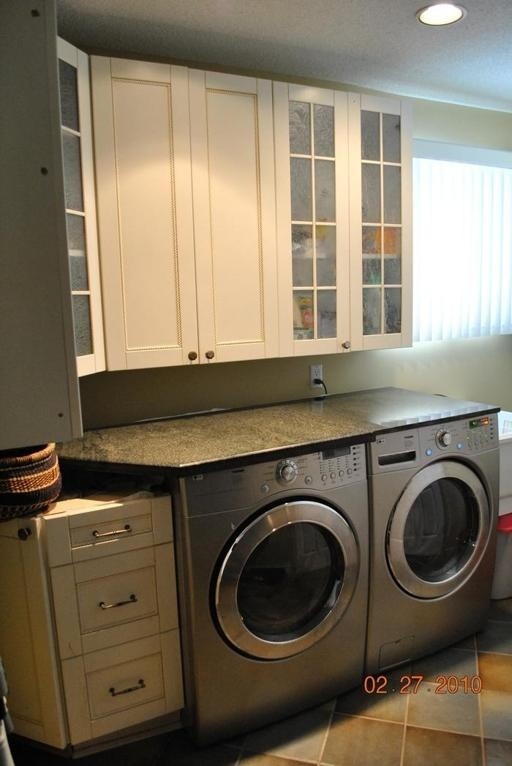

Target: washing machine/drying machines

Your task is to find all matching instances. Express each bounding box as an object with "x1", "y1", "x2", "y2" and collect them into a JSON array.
[
  {"x1": 175, "y1": 443, "x2": 370, "y2": 750},
  {"x1": 366, "y1": 412, "x2": 499, "y2": 677}
]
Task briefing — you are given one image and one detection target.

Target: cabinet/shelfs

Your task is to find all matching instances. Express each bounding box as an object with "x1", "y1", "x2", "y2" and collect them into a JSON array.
[
  {"x1": 0, "y1": 0, "x2": 82, "y2": 451},
  {"x1": 0, "y1": 484, "x2": 187, "y2": 760},
  {"x1": 271, "y1": 80, "x2": 413, "y2": 357},
  {"x1": 56, "y1": 34, "x2": 106, "y2": 376},
  {"x1": 92, "y1": 53, "x2": 282, "y2": 372}
]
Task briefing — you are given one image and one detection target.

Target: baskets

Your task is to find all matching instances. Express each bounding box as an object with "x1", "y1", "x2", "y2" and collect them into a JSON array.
[{"x1": 0, "y1": 442, "x2": 63, "y2": 519}]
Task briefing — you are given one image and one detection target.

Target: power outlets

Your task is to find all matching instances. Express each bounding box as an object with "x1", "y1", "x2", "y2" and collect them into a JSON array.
[{"x1": 310, "y1": 364, "x2": 323, "y2": 388}]
